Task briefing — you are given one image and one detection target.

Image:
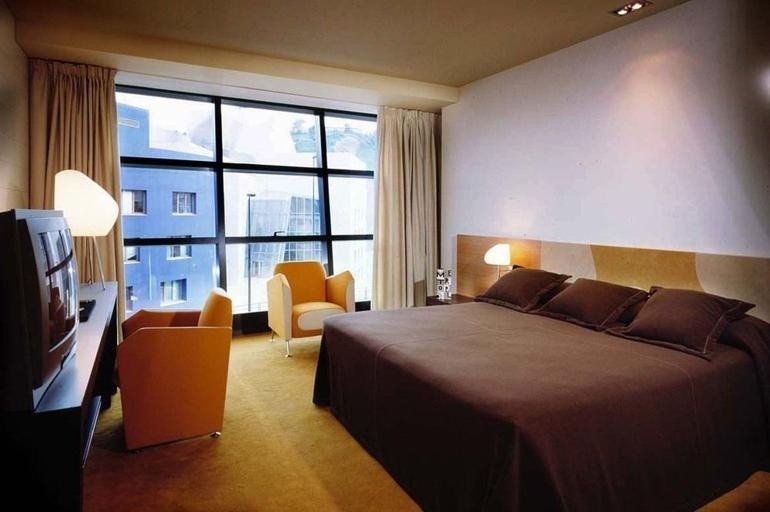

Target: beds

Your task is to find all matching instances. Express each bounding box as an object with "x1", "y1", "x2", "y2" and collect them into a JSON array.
[{"x1": 313, "y1": 282, "x2": 770, "y2": 512}]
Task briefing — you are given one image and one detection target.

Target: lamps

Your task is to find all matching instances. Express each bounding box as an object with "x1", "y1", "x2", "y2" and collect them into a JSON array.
[
  {"x1": 53, "y1": 170, "x2": 119, "y2": 290},
  {"x1": 611, "y1": 0, "x2": 653, "y2": 18},
  {"x1": 483, "y1": 243, "x2": 512, "y2": 281}
]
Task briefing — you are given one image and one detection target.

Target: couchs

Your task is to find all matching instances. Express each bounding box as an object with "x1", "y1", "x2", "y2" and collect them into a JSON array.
[
  {"x1": 115, "y1": 288, "x2": 234, "y2": 450},
  {"x1": 265, "y1": 260, "x2": 356, "y2": 358}
]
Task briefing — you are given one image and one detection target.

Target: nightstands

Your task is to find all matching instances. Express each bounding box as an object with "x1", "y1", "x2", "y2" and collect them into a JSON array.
[{"x1": 426, "y1": 295, "x2": 477, "y2": 307}]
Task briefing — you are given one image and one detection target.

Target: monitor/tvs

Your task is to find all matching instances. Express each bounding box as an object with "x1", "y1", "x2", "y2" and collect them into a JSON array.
[{"x1": 1, "y1": 209, "x2": 80, "y2": 409}]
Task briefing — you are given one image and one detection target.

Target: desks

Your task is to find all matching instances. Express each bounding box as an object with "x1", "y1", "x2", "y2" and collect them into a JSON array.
[{"x1": 35, "y1": 282, "x2": 118, "y2": 512}]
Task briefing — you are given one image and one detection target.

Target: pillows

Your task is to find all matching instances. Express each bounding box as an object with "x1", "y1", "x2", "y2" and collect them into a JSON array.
[
  {"x1": 529, "y1": 278, "x2": 647, "y2": 331},
  {"x1": 474, "y1": 264, "x2": 572, "y2": 313},
  {"x1": 607, "y1": 286, "x2": 758, "y2": 360}
]
